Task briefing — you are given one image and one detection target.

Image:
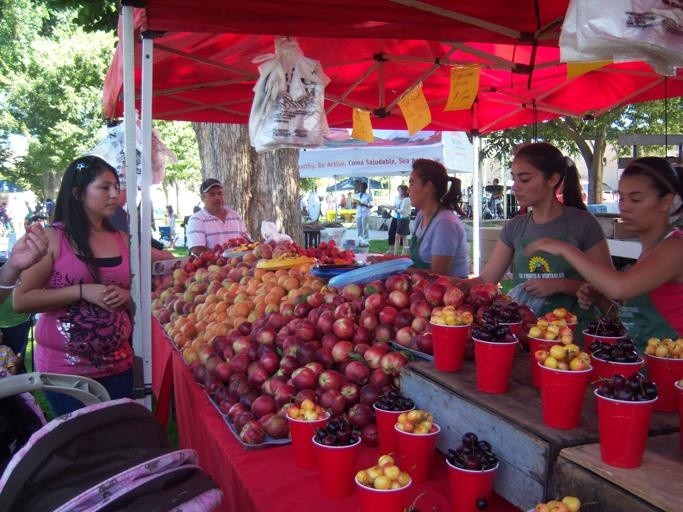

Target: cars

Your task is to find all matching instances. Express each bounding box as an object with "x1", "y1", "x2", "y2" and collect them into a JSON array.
[{"x1": 555, "y1": 182, "x2": 586, "y2": 203}]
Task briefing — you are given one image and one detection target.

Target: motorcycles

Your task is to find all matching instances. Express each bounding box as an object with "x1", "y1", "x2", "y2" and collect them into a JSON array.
[{"x1": 457, "y1": 185, "x2": 503, "y2": 221}]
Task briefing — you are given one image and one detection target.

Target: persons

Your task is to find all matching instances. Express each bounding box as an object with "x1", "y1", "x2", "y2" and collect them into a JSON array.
[
  {"x1": 302, "y1": 159, "x2": 469, "y2": 278},
  {"x1": 137, "y1": 178, "x2": 250, "y2": 260},
  {"x1": 425, "y1": 142, "x2": 683, "y2": 337},
  {"x1": 0, "y1": 153, "x2": 135, "y2": 477}
]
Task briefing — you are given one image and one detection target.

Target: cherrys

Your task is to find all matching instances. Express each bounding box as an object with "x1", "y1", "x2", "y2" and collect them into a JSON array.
[
  {"x1": 431, "y1": 307, "x2": 682, "y2": 402},
  {"x1": 288, "y1": 391, "x2": 580, "y2": 511}
]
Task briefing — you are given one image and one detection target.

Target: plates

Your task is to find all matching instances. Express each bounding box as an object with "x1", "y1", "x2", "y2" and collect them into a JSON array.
[
  {"x1": 311, "y1": 266, "x2": 360, "y2": 278},
  {"x1": 330, "y1": 258, "x2": 414, "y2": 290},
  {"x1": 258, "y1": 256, "x2": 317, "y2": 271},
  {"x1": 221, "y1": 247, "x2": 253, "y2": 257}
]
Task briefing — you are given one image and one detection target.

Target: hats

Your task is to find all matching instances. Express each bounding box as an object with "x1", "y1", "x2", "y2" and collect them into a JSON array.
[{"x1": 199, "y1": 178, "x2": 223, "y2": 193}]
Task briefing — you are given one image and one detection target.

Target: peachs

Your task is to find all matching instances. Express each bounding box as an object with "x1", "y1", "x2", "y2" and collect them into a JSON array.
[{"x1": 151, "y1": 238, "x2": 536, "y2": 443}]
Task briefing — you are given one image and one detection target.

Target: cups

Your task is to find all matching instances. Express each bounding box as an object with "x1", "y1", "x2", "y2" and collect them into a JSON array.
[
  {"x1": 429, "y1": 319, "x2": 471, "y2": 373},
  {"x1": 583, "y1": 327, "x2": 629, "y2": 352},
  {"x1": 445, "y1": 457, "x2": 500, "y2": 510},
  {"x1": 353, "y1": 464, "x2": 413, "y2": 512},
  {"x1": 591, "y1": 350, "x2": 645, "y2": 377},
  {"x1": 285, "y1": 411, "x2": 332, "y2": 471},
  {"x1": 393, "y1": 421, "x2": 441, "y2": 484},
  {"x1": 592, "y1": 385, "x2": 657, "y2": 470},
  {"x1": 644, "y1": 350, "x2": 682, "y2": 452},
  {"x1": 373, "y1": 402, "x2": 415, "y2": 456},
  {"x1": 473, "y1": 308, "x2": 589, "y2": 429},
  {"x1": 310, "y1": 435, "x2": 360, "y2": 500}
]
